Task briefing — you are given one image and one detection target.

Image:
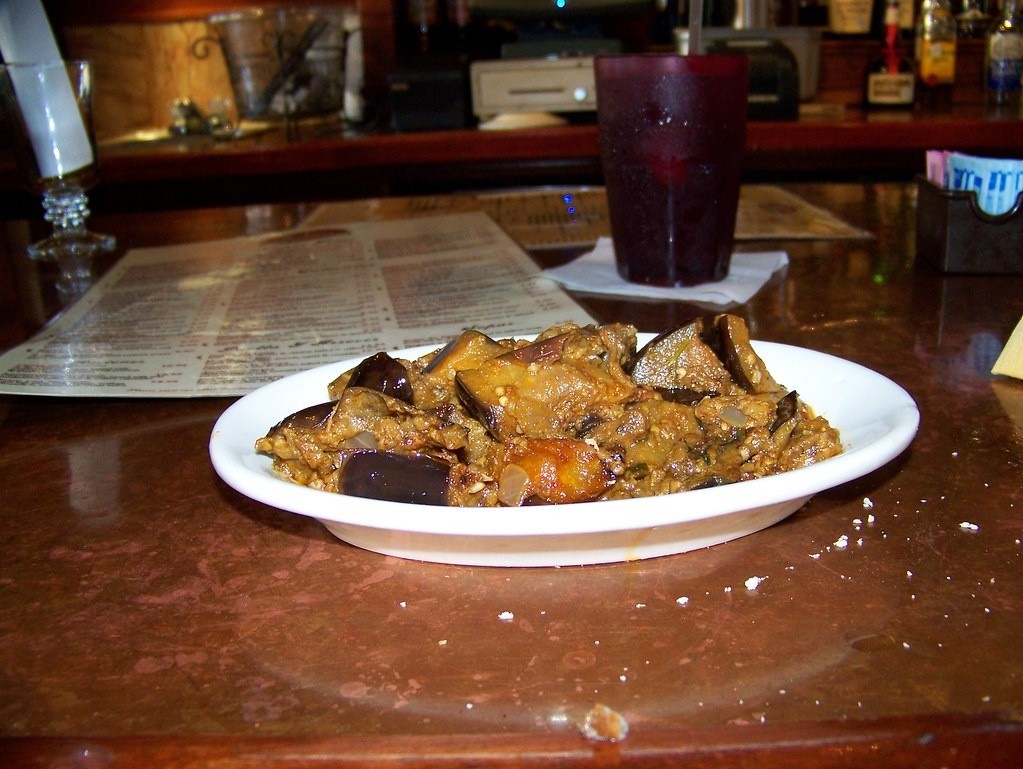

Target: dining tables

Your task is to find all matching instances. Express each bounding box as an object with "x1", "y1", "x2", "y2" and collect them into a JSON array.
[{"x1": 0, "y1": 182, "x2": 1023, "y2": 769}]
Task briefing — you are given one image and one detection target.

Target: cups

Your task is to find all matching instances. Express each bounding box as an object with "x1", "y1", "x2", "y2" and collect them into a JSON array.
[
  {"x1": 205, "y1": 3, "x2": 346, "y2": 137},
  {"x1": 592, "y1": 51, "x2": 753, "y2": 288}
]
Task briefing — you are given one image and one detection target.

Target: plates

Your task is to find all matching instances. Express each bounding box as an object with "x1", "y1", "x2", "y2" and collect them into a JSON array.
[{"x1": 209, "y1": 330, "x2": 920, "y2": 570}]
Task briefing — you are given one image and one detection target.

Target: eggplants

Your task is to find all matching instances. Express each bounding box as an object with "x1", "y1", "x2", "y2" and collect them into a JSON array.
[{"x1": 265, "y1": 309, "x2": 800, "y2": 506}]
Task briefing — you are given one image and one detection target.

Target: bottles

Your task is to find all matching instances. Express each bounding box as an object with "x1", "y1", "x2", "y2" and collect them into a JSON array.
[
  {"x1": 979, "y1": 0, "x2": 1023, "y2": 118},
  {"x1": 858, "y1": 0, "x2": 919, "y2": 123},
  {"x1": 826, "y1": 0, "x2": 874, "y2": 41},
  {"x1": 914, "y1": 0, "x2": 959, "y2": 115}
]
